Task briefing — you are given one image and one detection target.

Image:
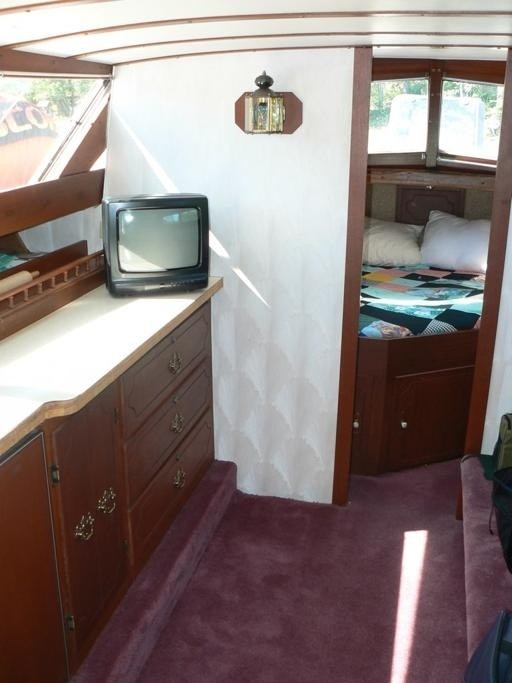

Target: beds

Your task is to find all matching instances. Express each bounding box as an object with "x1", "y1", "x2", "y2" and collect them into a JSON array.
[{"x1": 348, "y1": 166, "x2": 497, "y2": 474}]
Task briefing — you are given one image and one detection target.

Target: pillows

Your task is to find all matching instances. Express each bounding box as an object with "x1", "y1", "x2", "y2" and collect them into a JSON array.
[{"x1": 360, "y1": 209, "x2": 492, "y2": 275}]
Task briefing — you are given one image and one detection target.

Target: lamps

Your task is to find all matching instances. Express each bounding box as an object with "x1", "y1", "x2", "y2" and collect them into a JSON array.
[{"x1": 241, "y1": 69, "x2": 286, "y2": 136}]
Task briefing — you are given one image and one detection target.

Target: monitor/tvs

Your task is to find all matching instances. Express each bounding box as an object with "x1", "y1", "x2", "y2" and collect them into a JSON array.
[{"x1": 101, "y1": 194, "x2": 209, "y2": 297}]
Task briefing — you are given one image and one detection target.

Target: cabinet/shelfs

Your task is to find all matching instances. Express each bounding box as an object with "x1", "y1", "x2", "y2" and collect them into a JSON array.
[
  {"x1": 122, "y1": 299, "x2": 213, "y2": 578},
  {"x1": 58, "y1": 376, "x2": 134, "y2": 675},
  {"x1": 1, "y1": 427, "x2": 68, "y2": 682}
]
